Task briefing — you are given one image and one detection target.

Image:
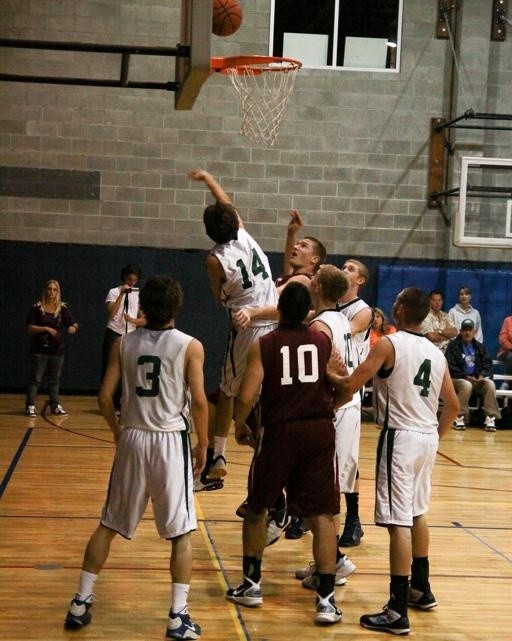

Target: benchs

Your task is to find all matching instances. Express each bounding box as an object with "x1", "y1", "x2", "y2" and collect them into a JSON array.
[{"x1": 360, "y1": 360, "x2": 512, "y2": 419}]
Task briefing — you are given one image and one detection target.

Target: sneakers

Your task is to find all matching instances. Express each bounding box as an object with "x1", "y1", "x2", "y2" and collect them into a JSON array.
[
  {"x1": 285, "y1": 516, "x2": 311, "y2": 540},
  {"x1": 49, "y1": 403, "x2": 69, "y2": 417},
  {"x1": 194, "y1": 469, "x2": 226, "y2": 491},
  {"x1": 295, "y1": 556, "x2": 355, "y2": 586},
  {"x1": 64, "y1": 599, "x2": 92, "y2": 630},
  {"x1": 24, "y1": 403, "x2": 37, "y2": 419},
  {"x1": 360, "y1": 602, "x2": 411, "y2": 635},
  {"x1": 484, "y1": 415, "x2": 497, "y2": 432},
  {"x1": 226, "y1": 574, "x2": 263, "y2": 607},
  {"x1": 302, "y1": 558, "x2": 347, "y2": 589},
  {"x1": 205, "y1": 456, "x2": 230, "y2": 480},
  {"x1": 338, "y1": 513, "x2": 365, "y2": 547},
  {"x1": 314, "y1": 589, "x2": 343, "y2": 624},
  {"x1": 453, "y1": 415, "x2": 467, "y2": 430},
  {"x1": 165, "y1": 606, "x2": 201, "y2": 640},
  {"x1": 264, "y1": 504, "x2": 298, "y2": 548},
  {"x1": 407, "y1": 580, "x2": 438, "y2": 612}
]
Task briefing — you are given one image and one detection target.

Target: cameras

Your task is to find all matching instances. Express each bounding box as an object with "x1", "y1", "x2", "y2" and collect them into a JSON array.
[{"x1": 124, "y1": 287, "x2": 139, "y2": 294}]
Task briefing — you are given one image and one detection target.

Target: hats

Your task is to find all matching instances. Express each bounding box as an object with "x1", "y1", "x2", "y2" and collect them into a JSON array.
[{"x1": 461, "y1": 319, "x2": 474, "y2": 331}]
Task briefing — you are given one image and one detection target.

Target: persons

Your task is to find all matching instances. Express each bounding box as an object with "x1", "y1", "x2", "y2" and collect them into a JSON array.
[
  {"x1": 105, "y1": 265, "x2": 149, "y2": 415},
  {"x1": 24, "y1": 281, "x2": 79, "y2": 417},
  {"x1": 62, "y1": 271, "x2": 209, "y2": 640},
  {"x1": 185, "y1": 168, "x2": 511, "y2": 635}
]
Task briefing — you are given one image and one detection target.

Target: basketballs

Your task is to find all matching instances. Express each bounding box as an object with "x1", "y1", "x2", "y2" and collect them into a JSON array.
[{"x1": 213, "y1": 0, "x2": 243, "y2": 35}]
